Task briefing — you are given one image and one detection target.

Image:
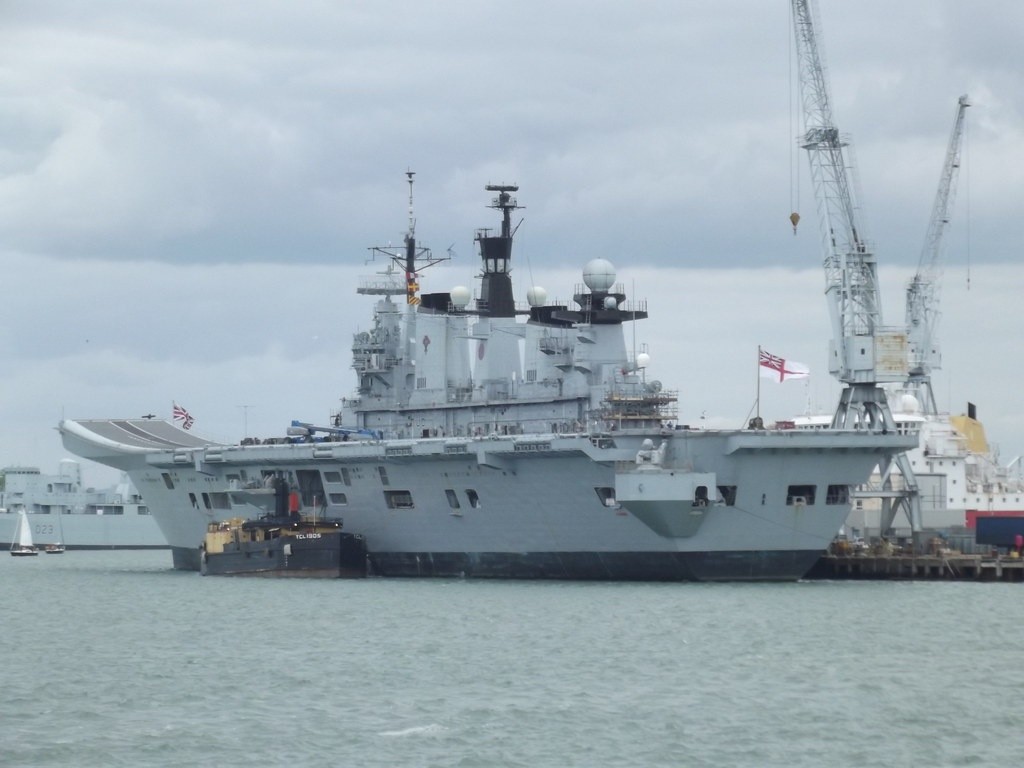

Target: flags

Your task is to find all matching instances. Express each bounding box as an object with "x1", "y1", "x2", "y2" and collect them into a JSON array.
[
  {"x1": 172, "y1": 403, "x2": 190, "y2": 421},
  {"x1": 405, "y1": 270, "x2": 424, "y2": 305},
  {"x1": 759, "y1": 350, "x2": 809, "y2": 383}
]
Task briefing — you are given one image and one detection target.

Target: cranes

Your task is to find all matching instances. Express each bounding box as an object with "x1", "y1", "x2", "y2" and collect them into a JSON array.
[
  {"x1": 793, "y1": 0, "x2": 912, "y2": 432},
  {"x1": 902, "y1": 90, "x2": 973, "y2": 423}
]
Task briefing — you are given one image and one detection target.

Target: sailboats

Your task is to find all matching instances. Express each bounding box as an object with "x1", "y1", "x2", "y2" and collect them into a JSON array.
[
  {"x1": 45, "y1": 505, "x2": 66, "y2": 554},
  {"x1": 9, "y1": 508, "x2": 38, "y2": 557}
]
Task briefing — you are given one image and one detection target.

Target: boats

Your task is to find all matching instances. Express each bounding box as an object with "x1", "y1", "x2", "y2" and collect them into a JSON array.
[
  {"x1": 52, "y1": 167, "x2": 927, "y2": 586},
  {"x1": 200, "y1": 476, "x2": 367, "y2": 580}
]
